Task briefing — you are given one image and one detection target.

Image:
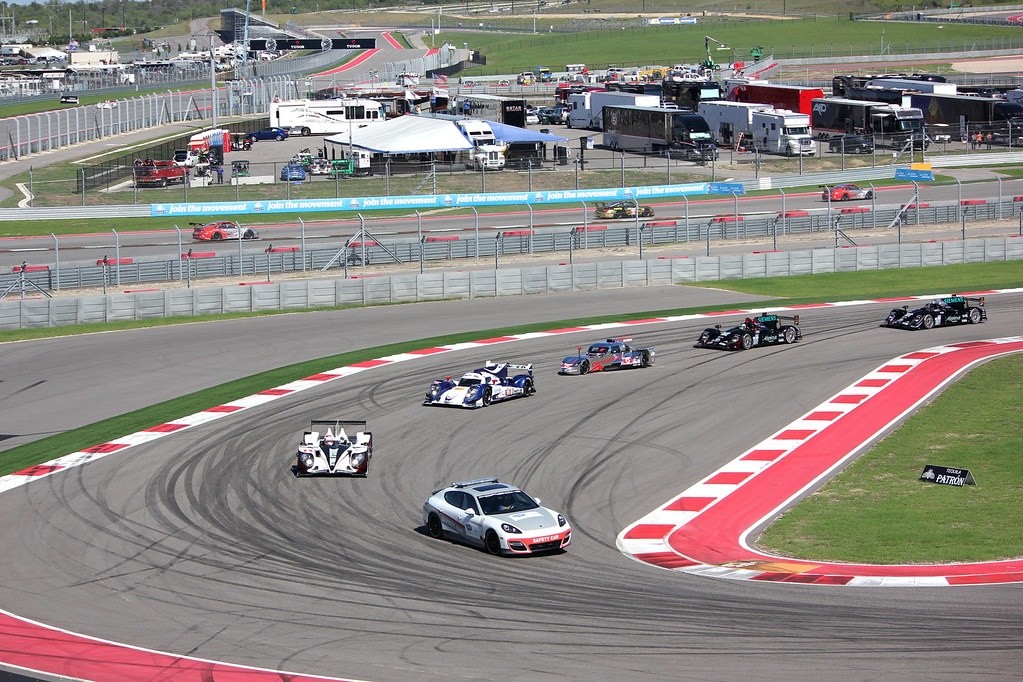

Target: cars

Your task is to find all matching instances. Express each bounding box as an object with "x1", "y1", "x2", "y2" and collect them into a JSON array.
[{"x1": 0, "y1": 27, "x2": 293, "y2": 95}]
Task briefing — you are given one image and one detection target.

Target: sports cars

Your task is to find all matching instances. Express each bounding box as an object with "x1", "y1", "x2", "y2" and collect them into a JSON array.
[
  {"x1": 420, "y1": 477, "x2": 574, "y2": 555},
  {"x1": 819, "y1": 180, "x2": 874, "y2": 201},
  {"x1": 98, "y1": 100, "x2": 120, "y2": 108},
  {"x1": 592, "y1": 201, "x2": 656, "y2": 220}
]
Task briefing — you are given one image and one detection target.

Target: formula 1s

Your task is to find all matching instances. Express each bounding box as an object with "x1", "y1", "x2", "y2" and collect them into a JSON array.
[
  {"x1": 292, "y1": 420, "x2": 373, "y2": 478},
  {"x1": 692, "y1": 312, "x2": 803, "y2": 350},
  {"x1": 560, "y1": 337, "x2": 656, "y2": 375},
  {"x1": 878, "y1": 293, "x2": 987, "y2": 331},
  {"x1": 424, "y1": 361, "x2": 537, "y2": 410}
]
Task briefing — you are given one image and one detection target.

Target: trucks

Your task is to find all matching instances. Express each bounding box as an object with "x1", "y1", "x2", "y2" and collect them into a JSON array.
[{"x1": 134, "y1": 62, "x2": 1023, "y2": 188}]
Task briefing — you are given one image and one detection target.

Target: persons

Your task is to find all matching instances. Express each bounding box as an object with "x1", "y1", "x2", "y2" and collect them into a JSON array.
[
  {"x1": 987, "y1": 131, "x2": 993, "y2": 151},
  {"x1": 233, "y1": 132, "x2": 240, "y2": 150},
  {"x1": 970, "y1": 132, "x2": 976, "y2": 151},
  {"x1": 429, "y1": 93, "x2": 437, "y2": 113},
  {"x1": 144, "y1": 157, "x2": 156, "y2": 169},
  {"x1": 216, "y1": 166, "x2": 224, "y2": 185},
  {"x1": 976, "y1": 131, "x2": 982, "y2": 150},
  {"x1": 464, "y1": 99, "x2": 471, "y2": 119},
  {"x1": 132, "y1": 157, "x2": 144, "y2": 169},
  {"x1": 413, "y1": 106, "x2": 421, "y2": 112},
  {"x1": 962, "y1": 131, "x2": 968, "y2": 150}
]
